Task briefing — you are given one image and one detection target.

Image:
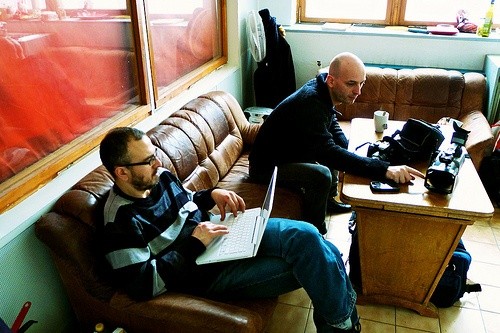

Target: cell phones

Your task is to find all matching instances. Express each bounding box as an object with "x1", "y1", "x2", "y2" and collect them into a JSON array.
[{"x1": 371, "y1": 181, "x2": 401, "y2": 192}]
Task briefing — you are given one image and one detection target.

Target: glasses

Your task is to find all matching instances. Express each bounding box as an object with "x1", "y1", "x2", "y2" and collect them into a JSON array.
[{"x1": 125, "y1": 148, "x2": 158, "y2": 167}]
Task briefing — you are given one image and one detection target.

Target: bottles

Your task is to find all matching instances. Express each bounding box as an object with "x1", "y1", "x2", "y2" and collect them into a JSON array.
[{"x1": 476, "y1": 0, "x2": 496, "y2": 37}]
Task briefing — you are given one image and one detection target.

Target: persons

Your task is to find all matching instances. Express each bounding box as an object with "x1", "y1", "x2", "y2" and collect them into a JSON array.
[
  {"x1": 100, "y1": 127, "x2": 362, "y2": 333},
  {"x1": 248, "y1": 52, "x2": 425, "y2": 235}
]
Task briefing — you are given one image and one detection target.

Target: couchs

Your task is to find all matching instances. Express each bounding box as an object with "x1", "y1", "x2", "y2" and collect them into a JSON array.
[
  {"x1": 33, "y1": 91, "x2": 304, "y2": 333},
  {"x1": 317, "y1": 67, "x2": 494, "y2": 171}
]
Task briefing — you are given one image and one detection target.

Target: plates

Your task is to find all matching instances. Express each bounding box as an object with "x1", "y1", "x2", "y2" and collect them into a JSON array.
[{"x1": 427, "y1": 26, "x2": 458, "y2": 35}]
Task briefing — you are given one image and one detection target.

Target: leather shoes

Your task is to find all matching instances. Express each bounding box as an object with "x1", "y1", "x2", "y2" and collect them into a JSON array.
[{"x1": 326, "y1": 197, "x2": 352, "y2": 216}]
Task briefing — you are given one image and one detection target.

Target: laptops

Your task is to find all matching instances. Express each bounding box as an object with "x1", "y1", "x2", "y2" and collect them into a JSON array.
[{"x1": 196, "y1": 166, "x2": 278, "y2": 265}]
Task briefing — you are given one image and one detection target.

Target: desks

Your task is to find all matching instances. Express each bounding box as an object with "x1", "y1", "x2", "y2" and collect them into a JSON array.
[
  {"x1": 340, "y1": 118, "x2": 496, "y2": 319},
  {"x1": 483, "y1": 55, "x2": 500, "y2": 167}
]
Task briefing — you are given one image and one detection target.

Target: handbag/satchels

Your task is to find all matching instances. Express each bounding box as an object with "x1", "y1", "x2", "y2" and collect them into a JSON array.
[
  {"x1": 349, "y1": 220, "x2": 482, "y2": 309},
  {"x1": 399, "y1": 118, "x2": 445, "y2": 162}
]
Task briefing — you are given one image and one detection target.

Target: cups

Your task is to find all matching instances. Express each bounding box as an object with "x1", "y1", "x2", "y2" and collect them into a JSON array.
[{"x1": 374, "y1": 110, "x2": 389, "y2": 133}]
